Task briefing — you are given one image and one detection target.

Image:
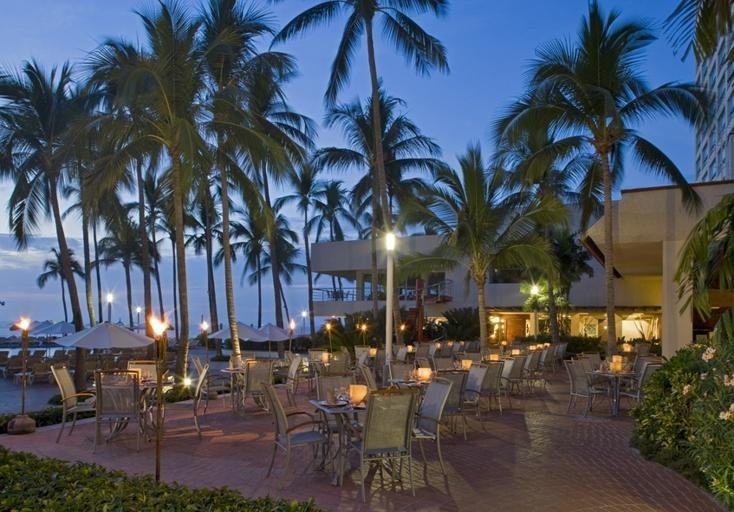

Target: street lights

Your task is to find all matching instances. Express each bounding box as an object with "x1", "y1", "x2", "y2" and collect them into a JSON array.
[
  {"x1": 384, "y1": 231, "x2": 396, "y2": 362},
  {"x1": 360, "y1": 322, "x2": 368, "y2": 347},
  {"x1": 14, "y1": 318, "x2": 32, "y2": 413},
  {"x1": 494, "y1": 317, "x2": 502, "y2": 344},
  {"x1": 200, "y1": 321, "x2": 212, "y2": 416},
  {"x1": 136, "y1": 306, "x2": 143, "y2": 334},
  {"x1": 106, "y1": 293, "x2": 116, "y2": 324},
  {"x1": 288, "y1": 318, "x2": 295, "y2": 353},
  {"x1": 529, "y1": 284, "x2": 540, "y2": 342},
  {"x1": 324, "y1": 323, "x2": 335, "y2": 355},
  {"x1": 300, "y1": 310, "x2": 307, "y2": 335},
  {"x1": 150, "y1": 316, "x2": 171, "y2": 482}
]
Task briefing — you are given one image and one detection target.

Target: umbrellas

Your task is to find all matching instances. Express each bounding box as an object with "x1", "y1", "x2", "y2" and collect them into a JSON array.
[
  {"x1": 28, "y1": 319, "x2": 155, "y2": 349},
  {"x1": 207, "y1": 321, "x2": 290, "y2": 357}
]
